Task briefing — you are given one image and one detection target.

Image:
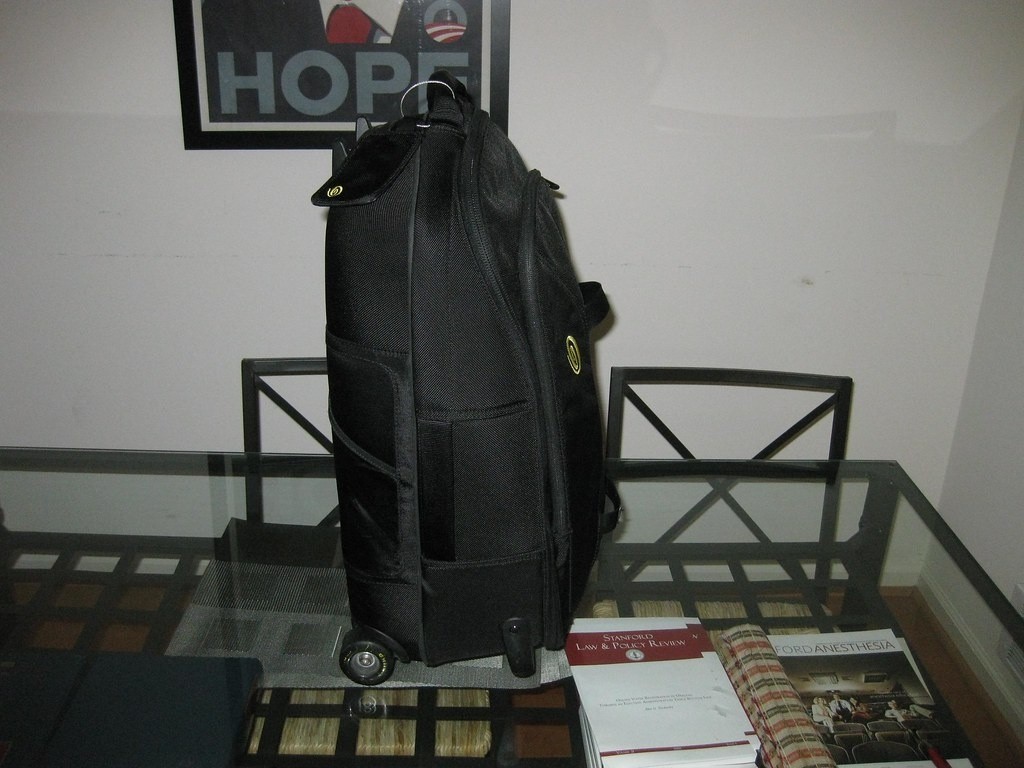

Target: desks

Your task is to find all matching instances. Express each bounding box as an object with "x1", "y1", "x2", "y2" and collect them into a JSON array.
[{"x1": 1, "y1": 445, "x2": 1024, "y2": 768}]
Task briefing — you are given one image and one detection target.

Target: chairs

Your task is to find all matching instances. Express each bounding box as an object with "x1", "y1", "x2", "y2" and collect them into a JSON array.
[
  {"x1": 240, "y1": 356, "x2": 495, "y2": 768},
  {"x1": 589, "y1": 364, "x2": 854, "y2": 642}
]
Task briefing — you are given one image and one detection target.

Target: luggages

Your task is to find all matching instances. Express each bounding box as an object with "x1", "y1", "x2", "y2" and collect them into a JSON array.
[{"x1": 309, "y1": 69, "x2": 624, "y2": 686}]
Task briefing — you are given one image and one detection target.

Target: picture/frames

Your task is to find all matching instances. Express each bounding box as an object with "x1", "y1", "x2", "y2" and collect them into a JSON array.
[{"x1": 172, "y1": 0, "x2": 511, "y2": 151}]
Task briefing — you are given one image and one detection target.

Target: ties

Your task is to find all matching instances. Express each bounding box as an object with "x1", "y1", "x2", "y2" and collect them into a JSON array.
[{"x1": 837, "y1": 701, "x2": 843, "y2": 711}]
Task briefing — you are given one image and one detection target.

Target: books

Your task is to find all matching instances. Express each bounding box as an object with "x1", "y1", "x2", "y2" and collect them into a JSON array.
[
  {"x1": 768, "y1": 628, "x2": 972, "y2": 768},
  {"x1": 565, "y1": 617, "x2": 761, "y2": 768}
]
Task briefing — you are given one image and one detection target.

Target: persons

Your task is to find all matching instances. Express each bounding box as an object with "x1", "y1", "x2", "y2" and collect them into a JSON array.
[
  {"x1": 848, "y1": 696, "x2": 870, "y2": 722},
  {"x1": 812, "y1": 697, "x2": 840, "y2": 734},
  {"x1": 829, "y1": 694, "x2": 855, "y2": 723},
  {"x1": 886, "y1": 699, "x2": 936, "y2": 725}
]
